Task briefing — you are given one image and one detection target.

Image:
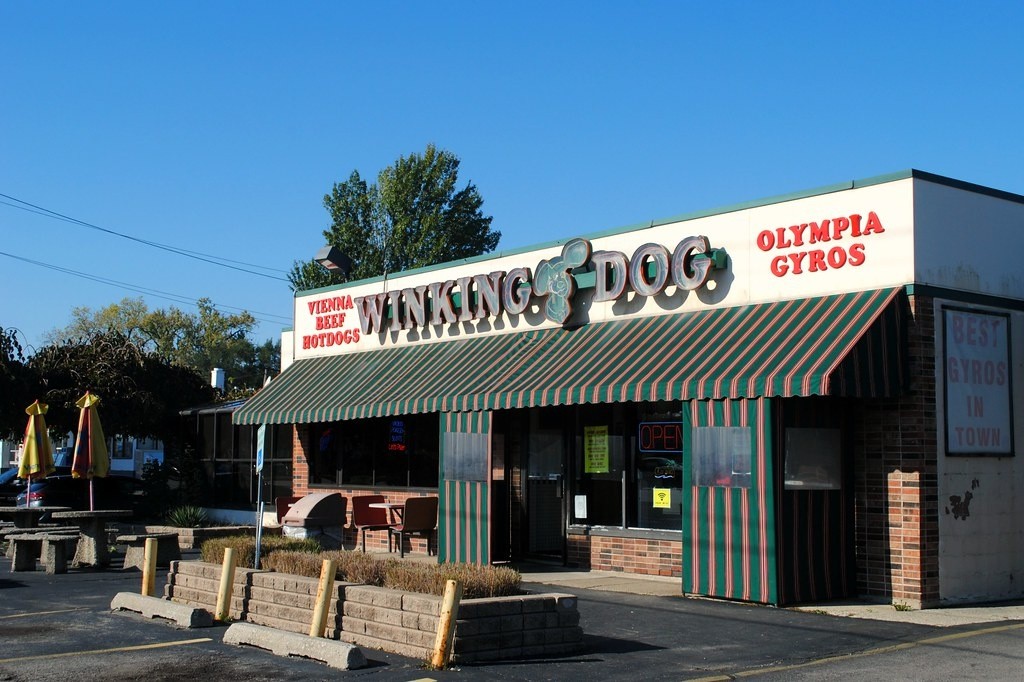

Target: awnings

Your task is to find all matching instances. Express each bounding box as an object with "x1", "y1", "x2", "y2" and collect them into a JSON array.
[{"x1": 231, "y1": 284, "x2": 907, "y2": 425}]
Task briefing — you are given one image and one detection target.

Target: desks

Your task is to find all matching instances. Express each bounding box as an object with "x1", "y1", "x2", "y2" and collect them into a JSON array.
[
  {"x1": 49, "y1": 510, "x2": 134, "y2": 570},
  {"x1": 0, "y1": 505, "x2": 74, "y2": 561}
]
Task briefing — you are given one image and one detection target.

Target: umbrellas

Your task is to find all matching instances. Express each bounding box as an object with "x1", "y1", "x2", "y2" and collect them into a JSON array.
[
  {"x1": 71, "y1": 389, "x2": 109, "y2": 512},
  {"x1": 16, "y1": 397, "x2": 56, "y2": 509}
]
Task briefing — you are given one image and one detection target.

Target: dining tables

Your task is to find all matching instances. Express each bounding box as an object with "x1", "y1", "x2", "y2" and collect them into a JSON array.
[{"x1": 368, "y1": 502, "x2": 404, "y2": 525}]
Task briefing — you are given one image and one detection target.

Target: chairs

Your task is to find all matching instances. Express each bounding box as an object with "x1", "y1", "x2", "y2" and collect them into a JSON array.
[
  {"x1": 388, "y1": 496, "x2": 439, "y2": 558},
  {"x1": 351, "y1": 494, "x2": 393, "y2": 554},
  {"x1": 275, "y1": 496, "x2": 305, "y2": 526}
]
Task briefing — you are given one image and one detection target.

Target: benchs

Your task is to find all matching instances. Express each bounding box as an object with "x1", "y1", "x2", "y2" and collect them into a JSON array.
[
  {"x1": 0, "y1": 523, "x2": 119, "y2": 575},
  {"x1": 116, "y1": 532, "x2": 182, "y2": 573}
]
